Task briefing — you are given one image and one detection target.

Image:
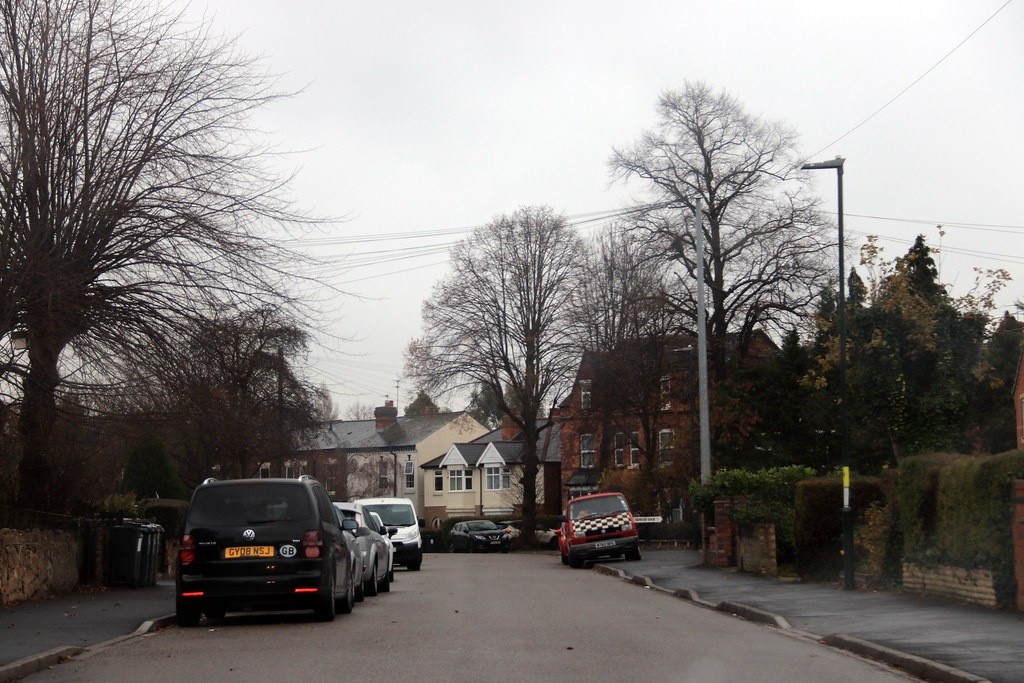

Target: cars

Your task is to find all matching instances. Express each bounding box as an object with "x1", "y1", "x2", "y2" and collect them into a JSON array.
[
  {"x1": 496, "y1": 520, "x2": 561, "y2": 549},
  {"x1": 553, "y1": 493, "x2": 640, "y2": 568},
  {"x1": 332, "y1": 503, "x2": 398, "y2": 600},
  {"x1": 448, "y1": 520, "x2": 511, "y2": 552}
]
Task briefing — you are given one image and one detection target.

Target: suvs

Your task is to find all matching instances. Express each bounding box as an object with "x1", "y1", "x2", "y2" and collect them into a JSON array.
[{"x1": 175, "y1": 474, "x2": 363, "y2": 626}]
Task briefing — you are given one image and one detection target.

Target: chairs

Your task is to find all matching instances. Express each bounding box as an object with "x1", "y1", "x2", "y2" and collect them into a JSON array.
[{"x1": 223, "y1": 501, "x2": 248, "y2": 524}]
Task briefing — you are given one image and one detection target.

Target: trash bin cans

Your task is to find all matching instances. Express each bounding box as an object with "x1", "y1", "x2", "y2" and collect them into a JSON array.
[{"x1": 106, "y1": 521, "x2": 166, "y2": 590}]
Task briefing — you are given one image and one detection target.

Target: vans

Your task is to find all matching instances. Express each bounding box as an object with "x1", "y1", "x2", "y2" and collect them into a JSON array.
[{"x1": 354, "y1": 498, "x2": 425, "y2": 570}]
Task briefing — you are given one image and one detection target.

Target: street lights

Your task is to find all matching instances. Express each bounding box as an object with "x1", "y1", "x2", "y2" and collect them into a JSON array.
[
  {"x1": 802, "y1": 159, "x2": 855, "y2": 589},
  {"x1": 673, "y1": 345, "x2": 694, "y2": 476}
]
